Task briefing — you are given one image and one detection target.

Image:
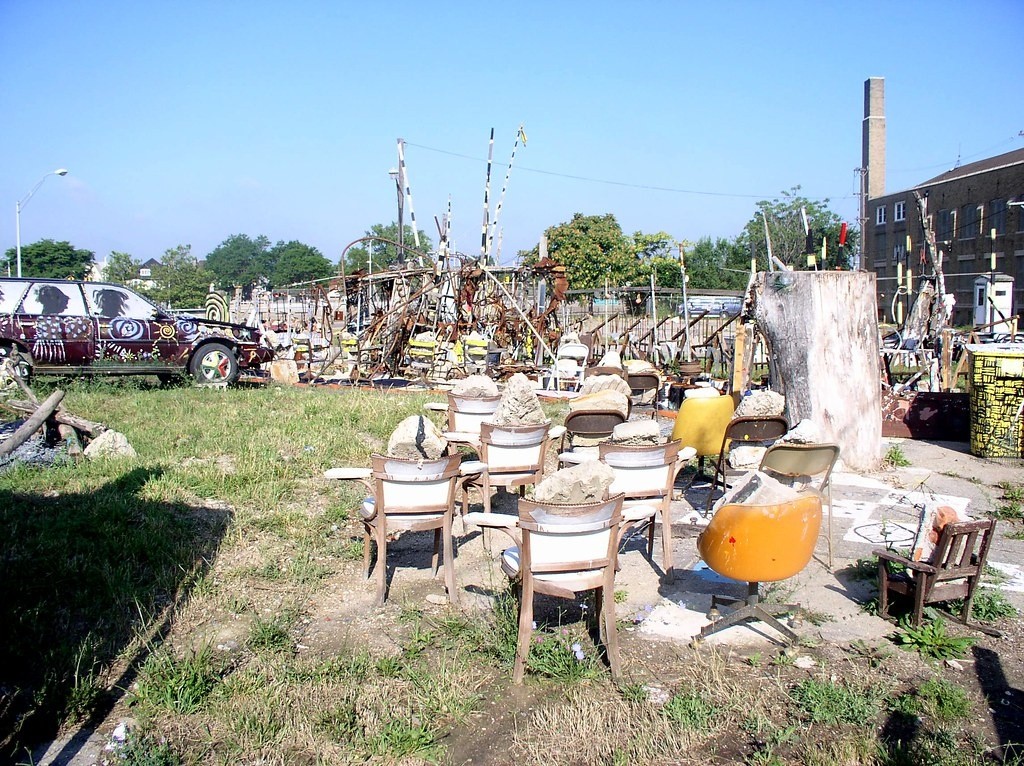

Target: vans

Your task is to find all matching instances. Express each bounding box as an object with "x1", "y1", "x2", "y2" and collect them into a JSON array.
[{"x1": 678, "y1": 294, "x2": 742, "y2": 317}]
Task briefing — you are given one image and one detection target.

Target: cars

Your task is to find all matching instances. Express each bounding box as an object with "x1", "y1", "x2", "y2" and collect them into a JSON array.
[{"x1": 0, "y1": 277, "x2": 274, "y2": 389}]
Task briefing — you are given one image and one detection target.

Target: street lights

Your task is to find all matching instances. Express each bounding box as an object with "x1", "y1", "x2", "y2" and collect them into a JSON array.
[{"x1": 17, "y1": 169, "x2": 67, "y2": 278}]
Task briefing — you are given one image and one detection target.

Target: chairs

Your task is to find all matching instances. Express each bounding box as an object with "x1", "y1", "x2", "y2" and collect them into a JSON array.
[{"x1": 360, "y1": 342, "x2": 1000, "y2": 687}]
